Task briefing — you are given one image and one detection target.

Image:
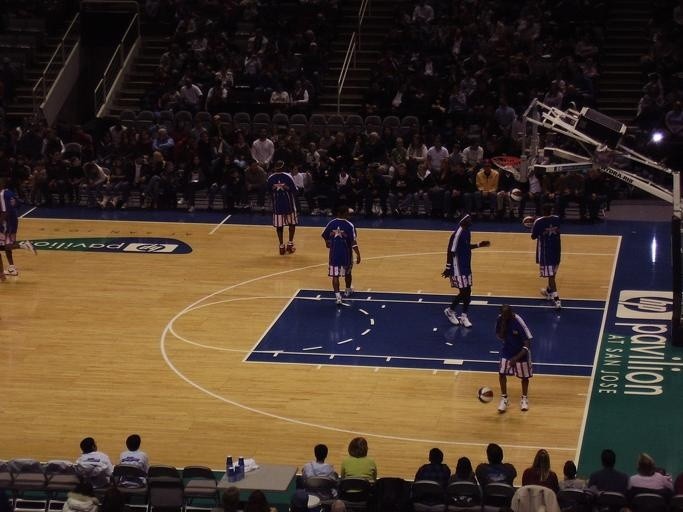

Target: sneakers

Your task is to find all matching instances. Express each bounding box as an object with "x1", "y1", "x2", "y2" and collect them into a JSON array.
[
  {"x1": 279, "y1": 239, "x2": 296, "y2": 255},
  {"x1": 443, "y1": 307, "x2": 472, "y2": 328},
  {"x1": 24, "y1": 239, "x2": 38, "y2": 256},
  {"x1": 3, "y1": 264, "x2": 19, "y2": 277},
  {"x1": 498, "y1": 394, "x2": 510, "y2": 413},
  {"x1": 519, "y1": 396, "x2": 530, "y2": 411},
  {"x1": 540, "y1": 288, "x2": 562, "y2": 309},
  {"x1": 334, "y1": 287, "x2": 354, "y2": 305}
]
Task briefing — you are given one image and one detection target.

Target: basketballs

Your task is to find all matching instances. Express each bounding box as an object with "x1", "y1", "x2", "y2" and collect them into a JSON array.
[
  {"x1": 478, "y1": 387, "x2": 493, "y2": 404},
  {"x1": 523, "y1": 217, "x2": 535, "y2": 229},
  {"x1": 510, "y1": 189, "x2": 522, "y2": 203}
]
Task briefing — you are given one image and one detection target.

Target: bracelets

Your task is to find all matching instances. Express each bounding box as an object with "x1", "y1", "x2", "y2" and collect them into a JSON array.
[
  {"x1": 446, "y1": 264, "x2": 451, "y2": 267},
  {"x1": 445, "y1": 267, "x2": 450, "y2": 270},
  {"x1": 476, "y1": 242, "x2": 479, "y2": 247},
  {"x1": 522, "y1": 346, "x2": 528, "y2": 352}
]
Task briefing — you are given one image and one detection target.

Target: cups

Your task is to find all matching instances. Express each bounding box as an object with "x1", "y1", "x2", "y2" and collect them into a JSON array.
[{"x1": 224, "y1": 455, "x2": 245, "y2": 483}]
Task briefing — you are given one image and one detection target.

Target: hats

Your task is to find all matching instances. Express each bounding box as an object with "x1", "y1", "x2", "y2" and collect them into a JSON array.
[{"x1": 483, "y1": 158, "x2": 493, "y2": 164}]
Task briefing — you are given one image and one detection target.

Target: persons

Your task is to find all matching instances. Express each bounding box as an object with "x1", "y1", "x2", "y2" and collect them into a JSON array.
[
  {"x1": 412, "y1": 443, "x2": 683, "y2": 512},
  {"x1": 494, "y1": 303, "x2": 534, "y2": 414},
  {"x1": 440, "y1": 214, "x2": 490, "y2": 329},
  {"x1": 265, "y1": 159, "x2": 299, "y2": 256},
  {"x1": 340, "y1": 437, "x2": 377, "y2": 488},
  {"x1": 529, "y1": 204, "x2": 562, "y2": 311},
  {"x1": 0, "y1": 252, "x2": 8, "y2": 282},
  {"x1": 330, "y1": 500, "x2": 346, "y2": 512},
  {"x1": 98, "y1": 488, "x2": 127, "y2": 511},
  {"x1": 299, "y1": 443, "x2": 338, "y2": 501},
  {"x1": 210, "y1": 487, "x2": 244, "y2": 512},
  {"x1": 243, "y1": 490, "x2": 277, "y2": 512},
  {"x1": 117, "y1": 434, "x2": 149, "y2": 489},
  {"x1": 289, "y1": 489, "x2": 309, "y2": 512},
  {"x1": 62, "y1": 482, "x2": 101, "y2": 512},
  {"x1": 0, "y1": 187, "x2": 37, "y2": 276},
  {"x1": 75, "y1": 437, "x2": 115, "y2": 488},
  {"x1": 320, "y1": 206, "x2": 362, "y2": 305}
]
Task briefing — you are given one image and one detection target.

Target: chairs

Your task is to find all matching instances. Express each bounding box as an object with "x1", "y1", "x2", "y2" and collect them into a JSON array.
[{"x1": 0, "y1": 459, "x2": 683, "y2": 512}]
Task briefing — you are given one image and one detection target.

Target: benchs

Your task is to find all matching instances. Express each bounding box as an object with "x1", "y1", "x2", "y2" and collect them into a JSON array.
[{"x1": 0, "y1": 2, "x2": 681, "y2": 211}]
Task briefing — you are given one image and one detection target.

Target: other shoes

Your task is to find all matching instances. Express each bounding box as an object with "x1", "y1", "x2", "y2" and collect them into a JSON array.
[
  {"x1": 296, "y1": 204, "x2": 608, "y2": 224},
  {"x1": 15, "y1": 192, "x2": 268, "y2": 212}
]
